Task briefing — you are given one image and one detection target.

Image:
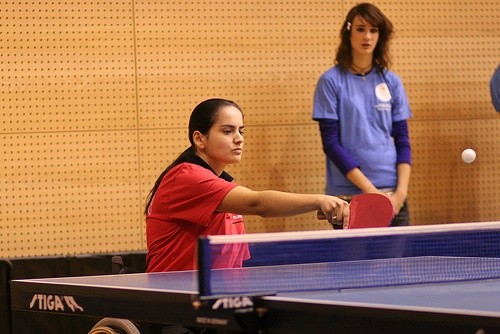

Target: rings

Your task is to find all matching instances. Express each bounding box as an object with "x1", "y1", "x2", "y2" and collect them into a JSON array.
[{"x1": 332, "y1": 216, "x2": 337, "y2": 220}]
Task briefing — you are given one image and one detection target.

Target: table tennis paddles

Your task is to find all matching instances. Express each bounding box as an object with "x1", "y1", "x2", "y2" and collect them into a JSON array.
[{"x1": 317, "y1": 193, "x2": 393, "y2": 230}]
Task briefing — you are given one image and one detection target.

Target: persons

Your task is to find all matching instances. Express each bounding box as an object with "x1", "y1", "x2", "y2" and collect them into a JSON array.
[
  {"x1": 313, "y1": 3, "x2": 412, "y2": 230},
  {"x1": 143, "y1": 98, "x2": 349, "y2": 273}
]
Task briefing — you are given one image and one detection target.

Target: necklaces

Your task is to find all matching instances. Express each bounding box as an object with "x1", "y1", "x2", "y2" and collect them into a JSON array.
[{"x1": 351, "y1": 63, "x2": 372, "y2": 80}]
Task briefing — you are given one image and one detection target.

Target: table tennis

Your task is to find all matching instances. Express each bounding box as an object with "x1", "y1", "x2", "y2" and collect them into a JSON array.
[{"x1": 462, "y1": 149, "x2": 476, "y2": 163}]
